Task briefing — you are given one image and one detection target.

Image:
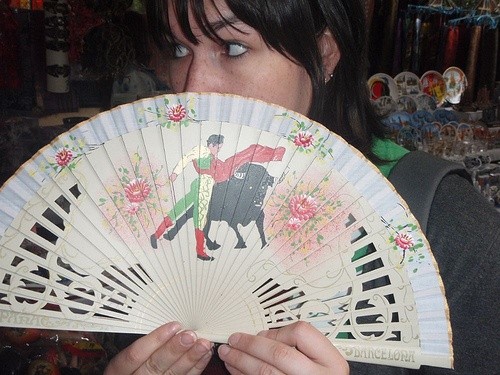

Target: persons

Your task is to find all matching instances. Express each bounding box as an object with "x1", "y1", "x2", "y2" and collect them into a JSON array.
[{"x1": 103, "y1": 0, "x2": 499, "y2": 375}]
